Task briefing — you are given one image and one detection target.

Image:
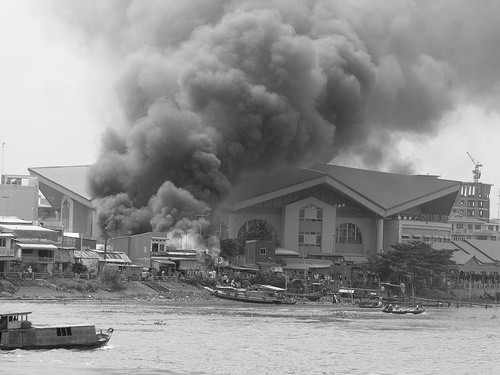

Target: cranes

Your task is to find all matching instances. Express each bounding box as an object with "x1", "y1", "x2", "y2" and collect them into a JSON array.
[{"x1": 467, "y1": 152, "x2": 482, "y2": 182}]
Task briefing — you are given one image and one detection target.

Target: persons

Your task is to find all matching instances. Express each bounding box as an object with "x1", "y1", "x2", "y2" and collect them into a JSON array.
[
  {"x1": 338, "y1": 295, "x2": 340, "y2": 303},
  {"x1": 142, "y1": 270, "x2": 147, "y2": 284},
  {"x1": 27, "y1": 266, "x2": 32, "y2": 273},
  {"x1": 334, "y1": 294, "x2": 337, "y2": 302},
  {"x1": 332, "y1": 295, "x2": 336, "y2": 304},
  {"x1": 160, "y1": 265, "x2": 254, "y2": 291}
]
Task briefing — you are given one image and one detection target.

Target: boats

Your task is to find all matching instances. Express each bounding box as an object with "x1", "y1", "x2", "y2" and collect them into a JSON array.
[
  {"x1": 0, "y1": 311, "x2": 114, "y2": 351},
  {"x1": 204, "y1": 285, "x2": 297, "y2": 305},
  {"x1": 383, "y1": 307, "x2": 425, "y2": 314}
]
